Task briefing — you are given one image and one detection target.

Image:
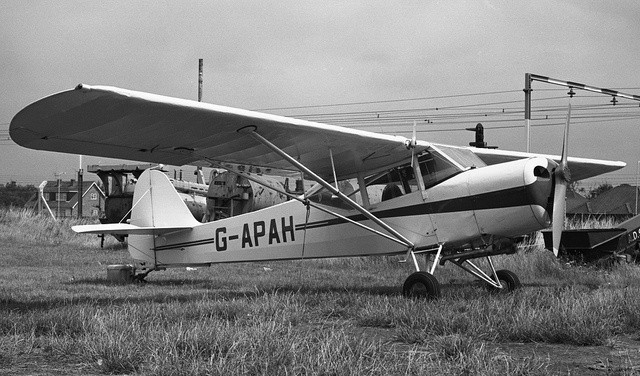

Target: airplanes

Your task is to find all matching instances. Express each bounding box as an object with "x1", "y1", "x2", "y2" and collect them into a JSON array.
[{"x1": 8, "y1": 80, "x2": 628, "y2": 303}]
